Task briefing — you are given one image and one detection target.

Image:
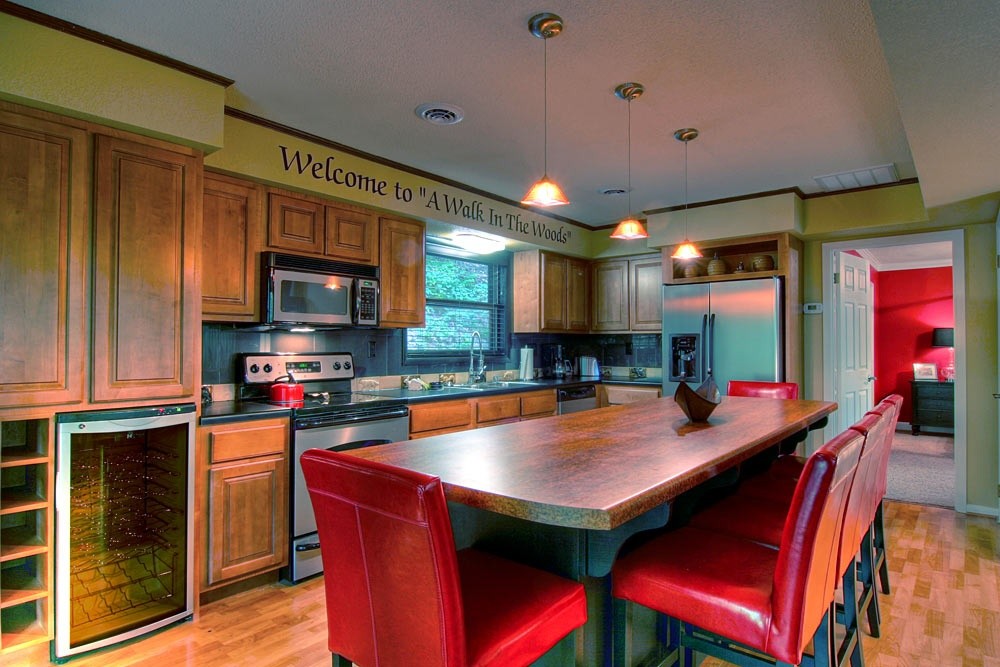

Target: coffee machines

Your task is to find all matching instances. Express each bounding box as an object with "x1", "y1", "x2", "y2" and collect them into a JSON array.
[{"x1": 550, "y1": 344, "x2": 568, "y2": 379}]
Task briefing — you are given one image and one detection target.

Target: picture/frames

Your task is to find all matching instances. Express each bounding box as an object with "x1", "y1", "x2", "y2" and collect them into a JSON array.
[{"x1": 912, "y1": 362, "x2": 940, "y2": 381}]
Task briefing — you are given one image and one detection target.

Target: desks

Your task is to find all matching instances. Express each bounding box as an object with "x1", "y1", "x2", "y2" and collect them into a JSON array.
[{"x1": 351, "y1": 395, "x2": 838, "y2": 666}]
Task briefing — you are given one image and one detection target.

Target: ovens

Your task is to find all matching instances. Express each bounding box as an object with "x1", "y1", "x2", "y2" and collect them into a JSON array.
[{"x1": 49, "y1": 402, "x2": 196, "y2": 663}]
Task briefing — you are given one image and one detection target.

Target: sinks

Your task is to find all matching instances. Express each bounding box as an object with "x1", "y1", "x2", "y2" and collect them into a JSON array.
[{"x1": 449, "y1": 380, "x2": 549, "y2": 391}]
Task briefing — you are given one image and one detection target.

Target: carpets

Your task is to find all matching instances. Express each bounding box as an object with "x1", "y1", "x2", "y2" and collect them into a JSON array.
[{"x1": 874, "y1": 428, "x2": 958, "y2": 513}]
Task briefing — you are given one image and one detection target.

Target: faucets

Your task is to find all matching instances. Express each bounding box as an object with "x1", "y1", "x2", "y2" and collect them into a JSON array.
[{"x1": 469, "y1": 330, "x2": 487, "y2": 384}]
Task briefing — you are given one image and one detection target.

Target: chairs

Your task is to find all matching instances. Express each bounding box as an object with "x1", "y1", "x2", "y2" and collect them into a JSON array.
[
  {"x1": 606, "y1": 394, "x2": 906, "y2": 667},
  {"x1": 723, "y1": 380, "x2": 802, "y2": 485},
  {"x1": 295, "y1": 448, "x2": 589, "y2": 666}
]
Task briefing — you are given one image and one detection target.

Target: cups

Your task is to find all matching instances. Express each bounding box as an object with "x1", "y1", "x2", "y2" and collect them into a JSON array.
[{"x1": 430, "y1": 382, "x2": 443, "y2": 389}]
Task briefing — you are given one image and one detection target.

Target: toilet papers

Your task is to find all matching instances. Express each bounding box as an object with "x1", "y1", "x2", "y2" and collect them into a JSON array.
[{"x1": 519, "y1": 347, "x2": 535, "y2": 383}]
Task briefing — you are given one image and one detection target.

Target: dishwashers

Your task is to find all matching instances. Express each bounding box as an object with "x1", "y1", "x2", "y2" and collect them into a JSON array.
[{"x1": 292, "y1": 412, "x2": 417, "y2": 582}]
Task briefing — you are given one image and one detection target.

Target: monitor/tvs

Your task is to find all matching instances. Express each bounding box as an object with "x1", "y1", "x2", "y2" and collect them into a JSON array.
[{"x1": 932, "y1": 328, "x2": 953, "y2": 346}]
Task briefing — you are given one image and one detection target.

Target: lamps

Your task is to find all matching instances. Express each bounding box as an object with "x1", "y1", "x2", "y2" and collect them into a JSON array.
[
  {"x1": 518, "y1": 13, "x2": 572, "y2": 209},
  {"x1": 932, "y1": 327, "x2": 959, "y2": 382},
  {"x1": 669, "y1": 126, "x2": 706, "y2": 261},
  {"x1": 609, "y1": 81, "x2": 653, "y2": 241}
]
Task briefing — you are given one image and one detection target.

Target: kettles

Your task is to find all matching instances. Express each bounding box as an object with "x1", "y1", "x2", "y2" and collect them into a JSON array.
[{"x1": 273, "y1": 372, "x2": 304, "y2": 402}]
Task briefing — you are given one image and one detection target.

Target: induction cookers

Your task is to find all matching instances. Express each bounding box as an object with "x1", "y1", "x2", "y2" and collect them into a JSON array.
[{"x1": 239, "y1": 349, "x2": 405, "y2": 421}]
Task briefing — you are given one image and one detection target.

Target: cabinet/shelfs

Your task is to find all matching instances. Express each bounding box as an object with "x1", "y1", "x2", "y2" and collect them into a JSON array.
[
  {"x1": 911, "y1": 381, "x2": 954, "y2": 436},
  {"x1": 202, "y1": 168, "x2": 430, "y2": 337},
  {"x1": 0, "y1": 100, "x2": 203, "y2": 665},
  {"x1": 511, "y1": 247, "x2": 664, "y2": 336}
]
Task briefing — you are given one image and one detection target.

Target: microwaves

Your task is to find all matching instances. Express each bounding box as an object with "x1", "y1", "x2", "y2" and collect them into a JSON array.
[{"x1": 230, "y1": 249, "x2": 381, "y2": 334}]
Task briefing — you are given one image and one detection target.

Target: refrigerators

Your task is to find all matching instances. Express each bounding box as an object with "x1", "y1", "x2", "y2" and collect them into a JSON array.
[{"x1": 659, "y1": 274, "x2": 787, "y2": 396}]
